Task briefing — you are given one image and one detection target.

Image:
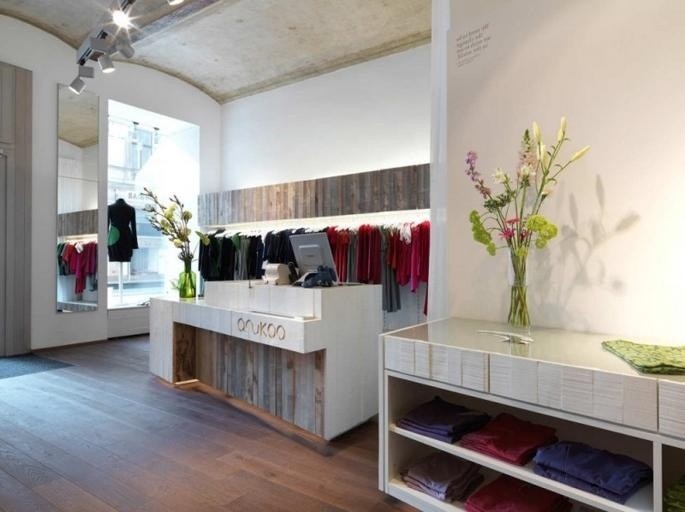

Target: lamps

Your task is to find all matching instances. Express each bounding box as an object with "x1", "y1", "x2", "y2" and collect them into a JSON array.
[{"x1": 67, "y1": 8, "x2": 135, "y2": 97}]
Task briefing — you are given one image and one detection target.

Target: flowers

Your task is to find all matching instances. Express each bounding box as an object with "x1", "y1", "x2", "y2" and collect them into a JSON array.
[
  {"x1": 461, "y1": 116, "x2": 593, "y2": 327},
  {"x1": 139, "y1": 188, "x2": 195, "y2": 287}
]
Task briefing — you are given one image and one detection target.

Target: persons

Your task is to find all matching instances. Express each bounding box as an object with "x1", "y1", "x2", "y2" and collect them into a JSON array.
[{"x1": 107, "y1": 198, "x2": 138, "y2": 262}]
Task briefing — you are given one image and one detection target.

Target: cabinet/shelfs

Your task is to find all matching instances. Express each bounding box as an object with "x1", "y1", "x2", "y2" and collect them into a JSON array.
[{"x1": 378, "y1": 315, "x2": 682, "y2": 512}]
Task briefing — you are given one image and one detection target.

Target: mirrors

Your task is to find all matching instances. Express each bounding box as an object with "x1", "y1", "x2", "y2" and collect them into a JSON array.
[{"x1": 57, "y1": 84, "x2": 100, "y2": 315}]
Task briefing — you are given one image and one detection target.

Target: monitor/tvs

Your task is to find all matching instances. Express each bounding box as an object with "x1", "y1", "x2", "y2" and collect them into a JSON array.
[{"x1": 289, "y1": 231, "x2": 339, "y2": 286}]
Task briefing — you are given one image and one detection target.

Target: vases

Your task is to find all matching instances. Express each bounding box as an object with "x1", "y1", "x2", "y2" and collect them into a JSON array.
[
  {"x1": 506, "y1": 284, "x2": 531, "y2": 327},
  {"x1": 178, "y1": 256, "x2": 197, "y2": 298}
]
